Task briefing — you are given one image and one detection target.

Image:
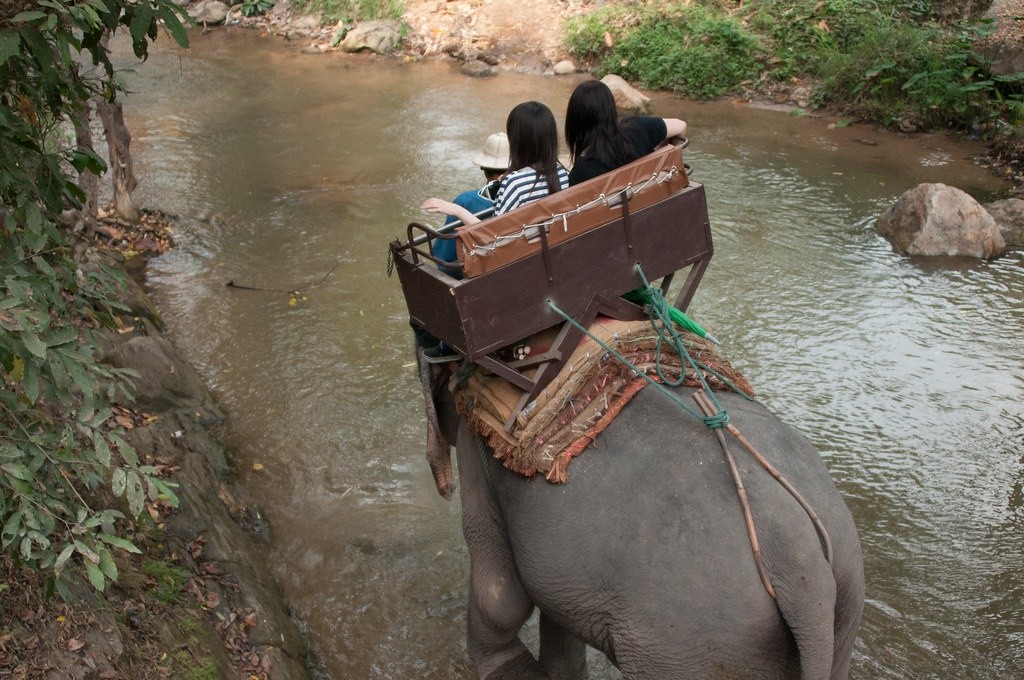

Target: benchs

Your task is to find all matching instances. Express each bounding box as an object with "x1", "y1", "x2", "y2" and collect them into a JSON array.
[{"x1": 379, "y1": 134, "x2": 717, "y2": 437}]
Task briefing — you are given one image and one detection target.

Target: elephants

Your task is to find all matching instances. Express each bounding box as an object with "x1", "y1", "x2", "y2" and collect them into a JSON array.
[{"x1": 416, "y1": 318, "x2": 865, "y2": 680}]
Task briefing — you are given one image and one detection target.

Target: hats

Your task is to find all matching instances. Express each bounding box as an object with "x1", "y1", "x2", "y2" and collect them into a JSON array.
[{"x1": 473, "y1": 133, "x2": 513, "y2": 168}]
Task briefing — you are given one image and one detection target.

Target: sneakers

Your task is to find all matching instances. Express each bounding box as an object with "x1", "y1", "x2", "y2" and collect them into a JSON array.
[{"x1": 422, "y1": 341, "x2": 464, "y2": 363}]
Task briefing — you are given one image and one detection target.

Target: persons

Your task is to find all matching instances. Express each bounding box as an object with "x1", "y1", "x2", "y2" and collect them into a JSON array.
[
  {"x1": 420, "y1": 101, "x2": 571, "y2": 280},
  {"x1": 564, "y1": 80, "x2": 688, "y2": 187}
]
